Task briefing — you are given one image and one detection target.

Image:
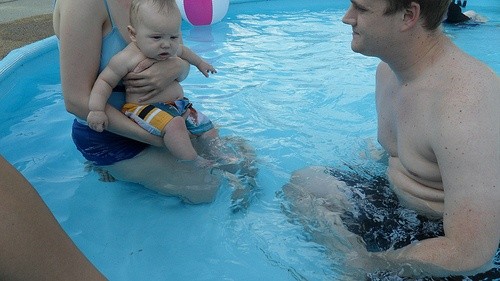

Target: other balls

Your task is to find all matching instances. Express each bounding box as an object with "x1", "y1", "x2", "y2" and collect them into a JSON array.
[{"x1": 176, "y1": 0, "x2": 229, "y2": 26}]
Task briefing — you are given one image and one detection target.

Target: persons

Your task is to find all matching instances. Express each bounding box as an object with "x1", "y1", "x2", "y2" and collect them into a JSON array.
[
  {"x1": 86, "y1": 1, "x2": 241, "y2": 173},
  {"x1": 51, "y1": 1, "x2": 260, "y2": 212},
  {"x1": 277, "y1": 0, "x2": 500, "y2": 281}
]
